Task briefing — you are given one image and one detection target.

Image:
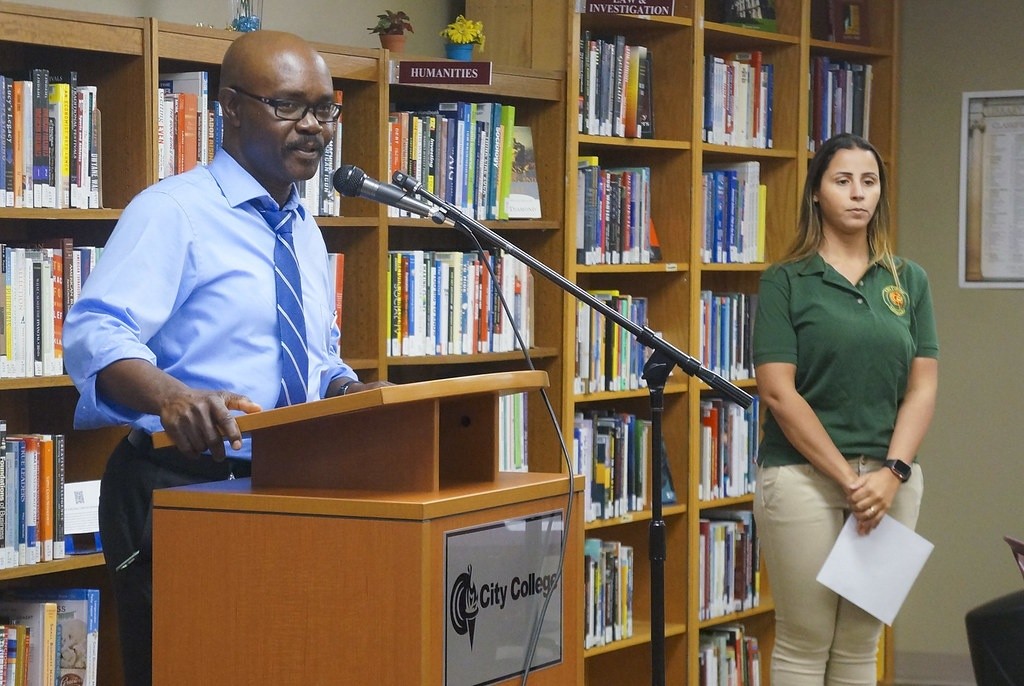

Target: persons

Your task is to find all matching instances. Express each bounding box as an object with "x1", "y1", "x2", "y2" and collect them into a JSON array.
[
  {"x1": 753, "y1": 134, "x2": 940, "y2": 686},
  {"x1": 63, "y1": 32, "x2": 396, "y2": 685}
]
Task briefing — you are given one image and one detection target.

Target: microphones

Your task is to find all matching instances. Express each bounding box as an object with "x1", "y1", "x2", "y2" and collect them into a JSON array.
[{"x1": 333, "y1": 165, "x2": 472, "y2": 236}]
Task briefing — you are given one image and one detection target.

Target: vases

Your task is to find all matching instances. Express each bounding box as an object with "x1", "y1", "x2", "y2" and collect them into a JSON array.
[{"x1": 443, "y1": 43, "x2": 473, "y2": 61}]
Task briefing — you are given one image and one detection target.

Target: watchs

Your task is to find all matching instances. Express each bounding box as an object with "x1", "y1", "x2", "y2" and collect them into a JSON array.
[{"x1": 883, "y1": 458, "x2": 912, "y2": 483}]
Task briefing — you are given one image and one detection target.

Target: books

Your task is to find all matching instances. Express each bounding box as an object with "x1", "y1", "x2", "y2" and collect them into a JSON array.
[
  {"x1": 0, "y1": 588, "x2": 99, "y2": 685},
  {"x1": 294, "y1": 90, "x2": 344, "y2": 219},
  {"x1": 705, "y1": 0, "x2": 778, "y2": 33},
  {"x1": 496, "y1": 392, "x2": 530, "y2": 473},
  {"x1": 699, "y1": 289, "x2": 759, "y2": 382},
  {"x1": 576, "y1": 30, "x2": 655, "y2": 139},
  {"x1": 388, "y1": 101, "x2": 542, "y2": 221},
  {"x1": 574, "y1": 289, "x2": 672, "y2": 395},
  {"x1": 875, "y1": 624, "x2": 885, "y2": 683},
  {"x1": 387, "y1": 251, "x2": 535, "y2": 356},
  {"x1": 699, "y1": 508, "x2": 761, "y2": 622},
  {"x1": 811, "y1": 0, "x2": 870, "y2": 45},
  {"x1": 157, "y1": 72, "x2": 226, "y2": 182},
  {"x1": 584, "y1": 538, "x2": 633, "y2": 651},
  {"x1": 808, "y1": 53, "x2": 872, "y2": 154},
  {"x1": 0, "y1": 419, "x2": 104, "y2": 571},
  {"x1": 576, "y1": 155, "x2": 662, "y2": 267},
  {"x1": 699, "y1": 396, "x2": 760, "y2": 504},
  {"x1": 703, "y1": 49, "x2": 774, "y2": 149},
  {"x1": 702, "y1": 161, "x2": 768, "y2": 265},
  {"x1": 0, "y1": 68, "x2": 102, "y2": 210},
  {"x1": 573, "y1": 406, "x2": 678, "y2": 524},
  {"x1": 328, "y1": 252, "x2": 344, "y2": 361},
  {"x1": 697, "y1": 624, "x2": 760, "y2": 686},
  {"x1": 0, "y1": 238, "x2": 104, "y2": 379}
]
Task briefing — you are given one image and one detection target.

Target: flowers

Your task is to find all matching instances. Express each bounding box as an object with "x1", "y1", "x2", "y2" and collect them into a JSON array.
[{"x1": 439, "y1": 15, "x2": 485, "y2": 53}]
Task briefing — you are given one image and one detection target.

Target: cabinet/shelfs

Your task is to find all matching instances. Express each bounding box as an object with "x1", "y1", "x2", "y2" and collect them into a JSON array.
[
  {"x1": 0, "y1": 0, "x2": 566, "y2": 686},
  {"x1": 466, "y1": 0, "x2": 902, "y2": 686}
]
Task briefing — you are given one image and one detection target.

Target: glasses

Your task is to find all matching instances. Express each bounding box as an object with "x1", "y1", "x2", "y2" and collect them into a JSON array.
[{"x1": 227, "y1": 85, "x2": 343, "y2": 123}]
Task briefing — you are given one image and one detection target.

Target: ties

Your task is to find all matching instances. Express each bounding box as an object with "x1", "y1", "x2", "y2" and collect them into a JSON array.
[{"x1": 245, "y1": 197, "x2": 309, "y2": 409}]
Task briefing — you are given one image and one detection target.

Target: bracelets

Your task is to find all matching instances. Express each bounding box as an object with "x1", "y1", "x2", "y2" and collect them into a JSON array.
[{"x1": 339, "y1": 380, "x2": 364, "y2": 395}]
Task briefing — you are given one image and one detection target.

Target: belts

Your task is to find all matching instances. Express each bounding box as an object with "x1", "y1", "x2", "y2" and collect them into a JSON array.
[{"x1": 126, "y1": 426, "x2": 251, "y2": 481}]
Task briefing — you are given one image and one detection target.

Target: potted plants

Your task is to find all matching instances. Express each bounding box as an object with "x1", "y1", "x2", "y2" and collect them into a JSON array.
[{"x1": 367, "y1": 10, "x2": 414, "y2": 52}]
